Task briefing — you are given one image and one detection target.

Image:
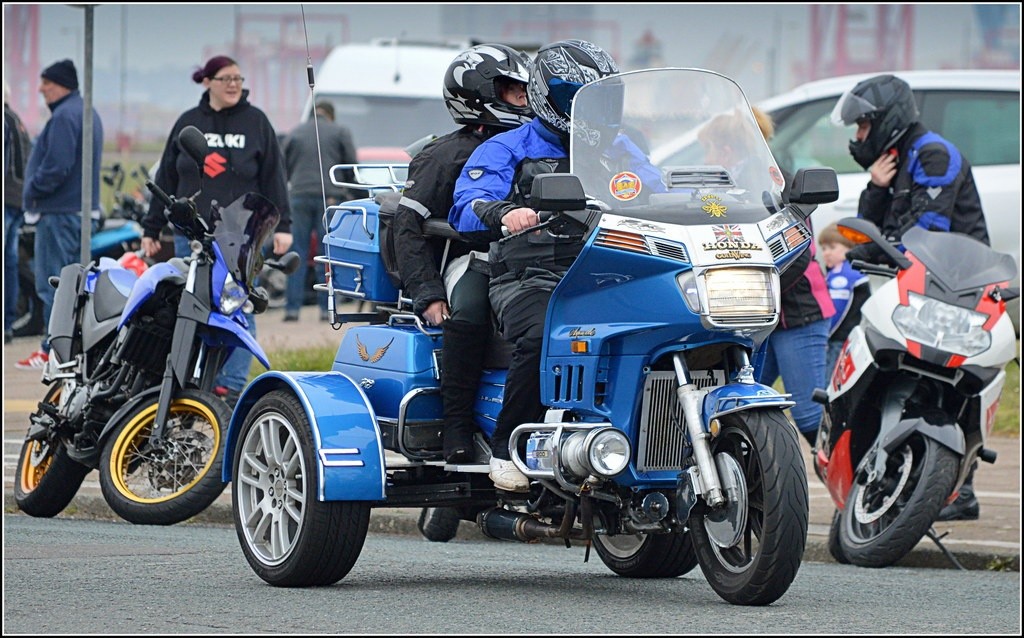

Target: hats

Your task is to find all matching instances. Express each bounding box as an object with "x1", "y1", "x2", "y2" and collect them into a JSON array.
[
  {"x1": 311, "y1": 102, "x2": 335, "y2": 121},
  {"x1": 41, "y1": 59, "x2": 77, "y2": 90}
]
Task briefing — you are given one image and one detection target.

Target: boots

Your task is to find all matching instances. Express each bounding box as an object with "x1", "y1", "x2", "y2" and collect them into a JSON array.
[{"x1": 442, "y1": 319, "x2": 485, "y2": 464}]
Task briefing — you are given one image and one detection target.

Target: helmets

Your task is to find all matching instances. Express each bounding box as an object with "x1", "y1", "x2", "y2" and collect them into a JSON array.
[
  {"x1": 526, "y1": 41, "x2": 625, "y2": 154},
  {"x1": 443, "y1": 43, "x2": 532, "y2": 129},
  {"x1": 842, "y1": 75, "x2": 918, "y2": 172}
]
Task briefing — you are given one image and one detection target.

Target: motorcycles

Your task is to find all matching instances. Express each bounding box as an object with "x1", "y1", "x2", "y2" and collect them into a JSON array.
[
  {"x1": 13, "y1": 130, "x2": 299, "y2": 526},
  {"x1": 808, "y1": 220, "x2": 1022, "y2": 569},
  {"x1": 219, "y1": 65, "x2": 845, "y2": 602}
]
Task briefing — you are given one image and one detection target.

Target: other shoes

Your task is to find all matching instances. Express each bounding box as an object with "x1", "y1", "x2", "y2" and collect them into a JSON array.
[
  {"x1": 320, "y1": 314, "x2": 331, "y2": 321},
  {"x1": 936, "y1": 487, "x2": 978, "y2": 521},
  {"x1": 282, "y1": 313, "x2": 299, "y2": 321}
]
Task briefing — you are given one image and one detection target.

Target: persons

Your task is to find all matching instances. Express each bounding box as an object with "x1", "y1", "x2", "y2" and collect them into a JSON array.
[
  {"x1": 819, "y1": 222, "x2": 868, "y2": 390},
  {"x1": 698, "y1": 107, "x2": 836, "y2": 448},
  {"x1": 393, "y1": 43, "x2": 537, "y2": 465},
  {"x1": 140, "y1": 55, "x2": 294, "y2": 410},
  {"x1": 15, "y1": 58, "x2": 102, "y2": 370},
  {"x1": 448, "y1": 38, "x2": 695, "y2": 493},
  {"x1": 4, "y1": 82, "x2": 31, "y2": 344},
  {"x1": 282, "y1": 101, "x2": 360, "y2": 322},
  {"x1": 829, "y1": 75, "x2": 992, "y2": 521}
]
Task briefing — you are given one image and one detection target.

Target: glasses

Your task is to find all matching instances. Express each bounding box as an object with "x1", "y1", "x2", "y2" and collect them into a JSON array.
[{"x1": 212, "y1": 77, "x2": 246, "y2": 85}]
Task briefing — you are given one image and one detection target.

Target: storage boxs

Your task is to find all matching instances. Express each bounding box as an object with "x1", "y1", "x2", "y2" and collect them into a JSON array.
[
  {"x1": 323, "y1": 197, "x2": 398, "y2": 304},
  {"x1": 332, "y1": 325, "x2": 445, "y2": 450}
]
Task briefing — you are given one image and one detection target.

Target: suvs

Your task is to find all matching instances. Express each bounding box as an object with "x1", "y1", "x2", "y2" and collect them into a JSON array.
[{"x1": 305, "y1": 37, "x2": 480, "y2": 152}]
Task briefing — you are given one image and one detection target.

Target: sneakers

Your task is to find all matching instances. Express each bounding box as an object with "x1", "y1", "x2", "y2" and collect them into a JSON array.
[
  {"x1": 488, "y1": 455, "x2": 531, "y2": 492},
  {"x1": 16, "y1": 353, "x2": 50, "y2": 369}
]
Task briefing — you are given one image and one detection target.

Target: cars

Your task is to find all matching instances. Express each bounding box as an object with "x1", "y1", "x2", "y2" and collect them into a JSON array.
[
  {"x1": 306, "y1": 144, "x2": 413, "y2": 303},
  {"x1": 647, "y1": 71, "x2": 1020, "y2": 299}
]
